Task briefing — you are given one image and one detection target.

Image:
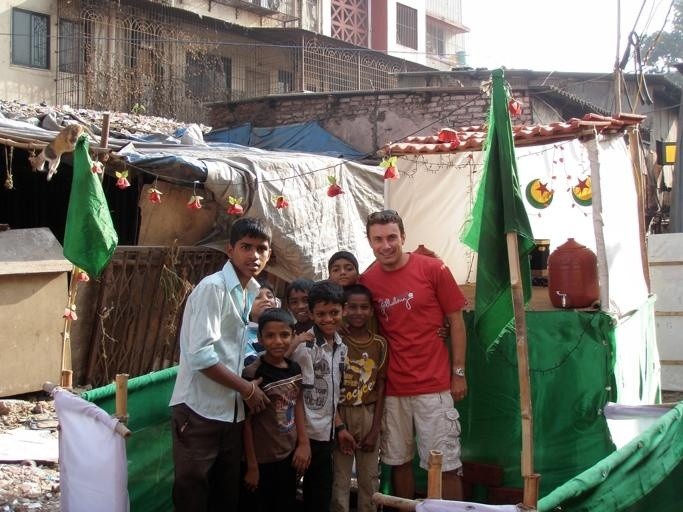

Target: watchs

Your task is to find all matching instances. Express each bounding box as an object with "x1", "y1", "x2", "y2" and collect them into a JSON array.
[{"x1": 451, "y1": 367, "x2": 465, "y2": 377}]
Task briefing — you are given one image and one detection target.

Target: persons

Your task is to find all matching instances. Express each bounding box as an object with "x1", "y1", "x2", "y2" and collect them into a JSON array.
[
  {"x1": 165, "y1": 217, "x2": 271, "y2": 512},
  {"x1": 354, "y1": 210, "x2": 470, "y2": 512}
]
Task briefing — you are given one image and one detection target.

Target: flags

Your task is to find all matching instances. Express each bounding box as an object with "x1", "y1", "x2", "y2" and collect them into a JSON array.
[
  {"x1": 60, "y1": 135, "x2": 121, "y2": 282},
  {"x1": 455, "y1": 70, "x2": 539, "y2": 390}
]
